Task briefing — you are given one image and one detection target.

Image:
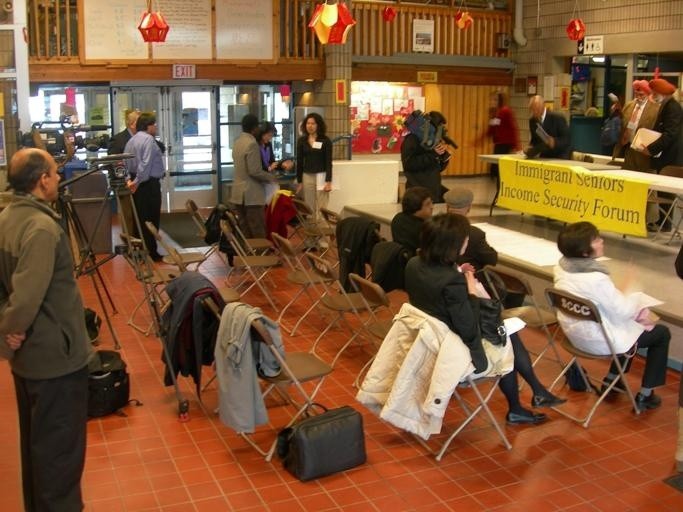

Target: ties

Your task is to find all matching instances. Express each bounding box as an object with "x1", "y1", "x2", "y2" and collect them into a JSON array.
[{"x1": 620, "y1": 104, "x2": 642, "y2": 145}]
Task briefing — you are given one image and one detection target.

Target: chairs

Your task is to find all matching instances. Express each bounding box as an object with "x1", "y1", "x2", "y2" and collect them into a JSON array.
[
  {"x1": 663, "y1": 195, "x2": 683, "y2": 246},
  {"x1": 622, "y1": 165, "x2": 683, "y2": 242}
]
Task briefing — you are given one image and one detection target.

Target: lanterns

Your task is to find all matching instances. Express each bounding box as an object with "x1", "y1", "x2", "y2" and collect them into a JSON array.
[
  {"x1": 382, "y1": 7, "x2": 396, "y2": 21},
  {"x1": 453, "y1": 10, "x2": 473, "y2": 33},
  {"x1": 137, "y1": 9, "x2": 170, "y2": 43},
  {"x1": 564, "y1": 17, "x2": 585, "y2": 42}
]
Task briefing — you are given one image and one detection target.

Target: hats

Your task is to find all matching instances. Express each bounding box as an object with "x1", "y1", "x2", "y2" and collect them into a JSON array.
[
  {"x1": 633, "y1": 80, "x2": 654, "y2": 98},
  {"x1": 441, "y1": 186, "x2": 474, "y2": 208},
  {"x1": 648, "y1": 78, "x2": 676, "y2": 95}
]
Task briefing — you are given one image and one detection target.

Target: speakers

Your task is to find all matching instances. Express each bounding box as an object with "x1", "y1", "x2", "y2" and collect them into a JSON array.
[{"x1": 497, "y1": 32, "x2": 511, "y2": 50}]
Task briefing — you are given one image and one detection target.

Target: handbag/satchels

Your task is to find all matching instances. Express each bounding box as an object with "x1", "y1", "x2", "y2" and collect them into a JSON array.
[{"x1": 276, "y1": 403, "x2": 367, "y2": 482}]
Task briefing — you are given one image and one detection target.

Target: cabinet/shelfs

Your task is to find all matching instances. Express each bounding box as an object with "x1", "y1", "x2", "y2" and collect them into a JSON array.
[{"x1": 570, "y1": 77, "x2": 594, "y2": 115}]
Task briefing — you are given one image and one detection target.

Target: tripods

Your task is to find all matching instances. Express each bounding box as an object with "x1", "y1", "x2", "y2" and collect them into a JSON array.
[
  {"x1": 56, "y1": 196, "x2": 123, "y2": 351},
  {"x1": 76, "y1": 187, "x2": 192, "y2": 413}
]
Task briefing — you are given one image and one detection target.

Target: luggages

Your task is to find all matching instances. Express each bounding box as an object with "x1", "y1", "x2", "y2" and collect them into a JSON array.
[{"x1": 89, "y1": 350, "x2": 131, "y2": 417}]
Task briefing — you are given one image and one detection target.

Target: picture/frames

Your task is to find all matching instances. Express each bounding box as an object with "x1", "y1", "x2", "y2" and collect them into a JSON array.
[
  {"x1": 527, "y1": 77, "x2": 537, "y2": 96},
  {"x1": 515, "y1": 78, "x2": 525, "y2": 92}
]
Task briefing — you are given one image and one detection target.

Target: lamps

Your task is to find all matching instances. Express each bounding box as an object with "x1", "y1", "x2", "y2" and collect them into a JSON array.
[
  {"x1": 138, "y1": 0, "x2": 169, "y2": 42},
  {"x1": 565, "y1": 0, "x2": 585, "y2": 41},
  {"x1": 454, "y1": 0, "x2": 474, "y2": 32},
  {"x1": 382, "y1": 3, "x2": 396, "y2": 23}
]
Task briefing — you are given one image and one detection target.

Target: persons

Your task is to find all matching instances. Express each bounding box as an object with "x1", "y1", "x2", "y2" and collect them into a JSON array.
[
  {"x1": 0, "y1": 146, "x2": 96, "y2": 511},
  {"x1": 121, "y1": 112, "x2": 165, "y2": 264},
  {"x1": 585, "y1": 76, "x2": 682, "y2": 177},
  {"x1": 550, "y1": 220, "x2": 671, "y2": 415},
  {"x1": 103, "y1": 108, "x2": 166, "y2": 241},
  {"x1": 293, "y1": 112, "x2": 332, "y2": 221},
  {"x1": 425, "y1": 187, "x2": 497, "y2": 266},
  {"x1": 526, "y1": 91, "x2": 568, "y2": 163},
  {"x1": 402, "y1": 211, "x2": 568, "y2": 426},
  {"x1": 387, "y1": 186, "x2": 433, "y2": 248},
  {"x1": 397, "y1": 108, "x2": 449, "y2": 202},
  {"x1": 467, "y1": 90, "x2": 522, "y2": 180},
  {"x1": 252, "y1": 121, "x2": 277, "y2": 171},
  {"x1": 226, "y1": 114, "x2": 272, "y2": 241},
  {"x1": 673, "y1": 243, "x2": 683, "y2": 476}
]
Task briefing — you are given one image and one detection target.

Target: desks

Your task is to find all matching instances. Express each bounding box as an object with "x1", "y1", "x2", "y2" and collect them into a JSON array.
[{"x1": 476, "y1": 153, "x2": 683, "y2": 245}]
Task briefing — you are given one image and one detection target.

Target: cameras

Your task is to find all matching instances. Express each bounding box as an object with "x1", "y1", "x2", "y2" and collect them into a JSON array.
[{"x1": 106, "y1": 160, "x2": 131, "y2": 184}]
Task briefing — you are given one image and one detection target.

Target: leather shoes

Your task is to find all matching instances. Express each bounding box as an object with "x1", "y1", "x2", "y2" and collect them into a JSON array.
[
  {"x1": 505, "y1": 410, "x2": 547, "y2": 426},
  {"x1": 634, "y1": 392, "x2": 663, "y2": 411},
  {"x1": 530, "y1": 394, "x2": 570, "y2": 408},
  {"x1": 599, "y1": 377, "x2": 629, "y2": 395}
]
